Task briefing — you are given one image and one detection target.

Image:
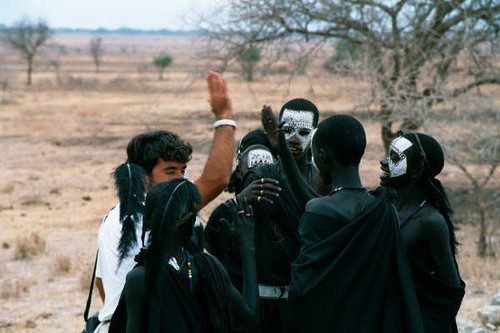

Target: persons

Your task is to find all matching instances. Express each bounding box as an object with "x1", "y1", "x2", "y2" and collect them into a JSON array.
[{"x1": 82, "y1": 69, "x2": 465, "y2": 333}]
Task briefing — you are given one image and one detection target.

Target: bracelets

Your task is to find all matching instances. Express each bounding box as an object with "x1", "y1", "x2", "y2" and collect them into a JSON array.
[{"x1": 213, "y1": 119, "x2": 237, "y2": 130}]
